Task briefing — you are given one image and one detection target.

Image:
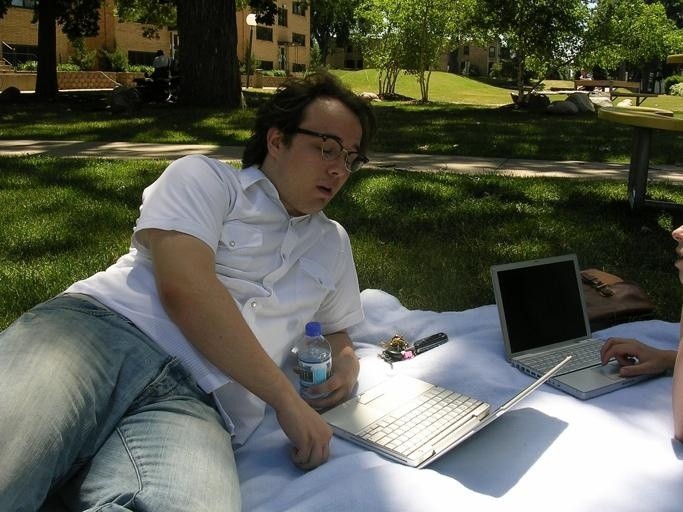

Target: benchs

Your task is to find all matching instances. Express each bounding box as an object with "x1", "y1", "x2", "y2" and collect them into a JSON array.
[
  {"x1": 549, "y1": 87, "x2": 590, "y2": 100},
  {"x1": 612, "y1": 92, "x2": 658, "y2": 106},
  {"x1": 597, "y1": 107, "x2": 683, "y2": 219}
]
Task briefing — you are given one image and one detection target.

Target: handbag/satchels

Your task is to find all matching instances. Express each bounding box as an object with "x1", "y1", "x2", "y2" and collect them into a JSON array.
[{"x1": 576, "y1": 266, "x2": 657, "y2": 329}]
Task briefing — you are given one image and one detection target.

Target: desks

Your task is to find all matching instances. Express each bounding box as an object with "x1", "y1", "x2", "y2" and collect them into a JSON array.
[
  {"x1": 667, "y1": 53, "x2": 683, "y2": 65},
  {"x1": 573, "y1": 80, "x2": 641, "y2": 106}
]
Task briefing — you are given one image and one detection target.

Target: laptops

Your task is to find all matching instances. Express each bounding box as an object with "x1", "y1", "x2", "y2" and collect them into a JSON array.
[
  {"x1": 319, "y1": 355, "x2": 572, "y2": 469},
  {"x1": 490, "y1": 252, "x2": 666, "y2": 399}
]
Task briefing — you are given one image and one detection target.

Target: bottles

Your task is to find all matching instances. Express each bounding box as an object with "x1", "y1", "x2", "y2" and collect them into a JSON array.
[{"x1": 296, "y1": 321, "x2": 334, "y2": 414}]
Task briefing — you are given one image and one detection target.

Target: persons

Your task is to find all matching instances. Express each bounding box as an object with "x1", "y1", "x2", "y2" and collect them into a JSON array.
[
  {"x1": 599, "y1": 221, "x2": 683, "y2": 442},
  {"x1": 0, "y1": 71, "x2": 377, "y2": 511},
  {"x1": 144, "y1": 49, "x2": 180, "y2": 104}
]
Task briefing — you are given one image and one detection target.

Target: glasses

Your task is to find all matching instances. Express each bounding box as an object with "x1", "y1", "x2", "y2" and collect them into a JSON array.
[{"x1": 286, "y1": 126, "x2": 369, "y2": 174}]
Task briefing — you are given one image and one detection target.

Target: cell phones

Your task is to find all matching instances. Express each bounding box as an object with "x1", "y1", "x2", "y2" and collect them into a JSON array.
[{"x1": 405, "y1": 332, "x2": 448, "y2": 354}]
